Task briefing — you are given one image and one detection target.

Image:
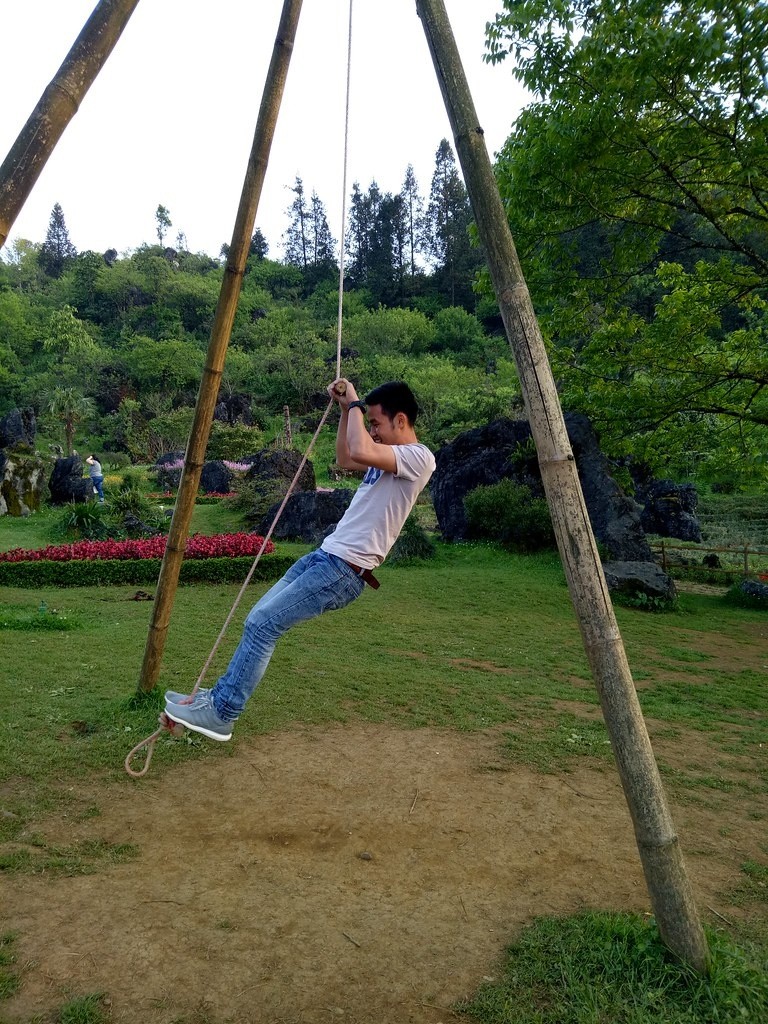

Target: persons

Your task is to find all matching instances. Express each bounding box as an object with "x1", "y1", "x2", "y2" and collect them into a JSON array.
[
  {"x1": 85, "y1": 453, "x2": 107, "y2": 501},
  {"x1": 164, "y1": 373, "x2": 437, "y2": 742}
]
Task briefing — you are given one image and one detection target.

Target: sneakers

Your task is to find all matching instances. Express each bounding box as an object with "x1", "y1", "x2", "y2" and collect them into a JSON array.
[
  {"x1": 165, "y1": 687, "x2": 213, "y2": 706},
  {"x1": 164, "y1": 696, "x2": 238, "y2": 742}
]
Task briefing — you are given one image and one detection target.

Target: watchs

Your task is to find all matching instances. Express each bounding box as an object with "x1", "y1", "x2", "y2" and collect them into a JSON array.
[{"x1": 346, "y1": 399, "x2": 371, "y2": 414}]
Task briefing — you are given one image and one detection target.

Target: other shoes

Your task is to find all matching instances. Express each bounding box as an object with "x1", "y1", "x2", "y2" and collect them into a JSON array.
[{"x1": 97, "y1": 500, "x2": 103, "y2": 503}]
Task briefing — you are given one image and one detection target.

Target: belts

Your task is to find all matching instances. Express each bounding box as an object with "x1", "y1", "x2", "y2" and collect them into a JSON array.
[{"x1": 344, "y1": 559, "x2": 380, "y2": 590}]
